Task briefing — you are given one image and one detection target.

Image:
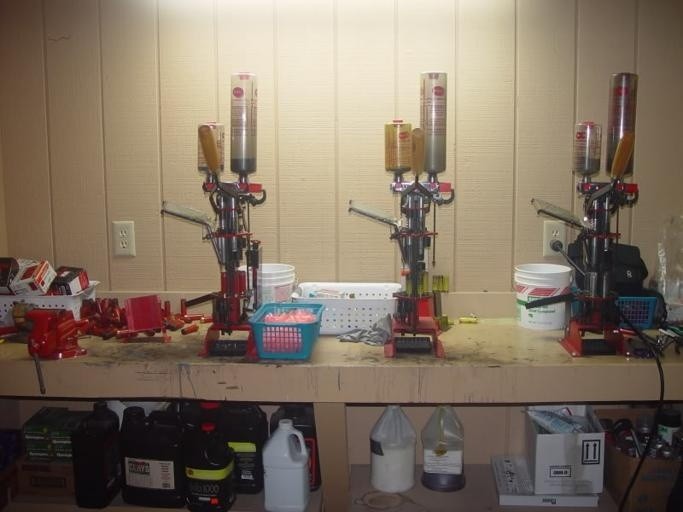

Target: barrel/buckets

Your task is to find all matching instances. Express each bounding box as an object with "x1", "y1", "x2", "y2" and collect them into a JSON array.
[
  {"x1": 370, "y1": 404, "x2": 417, "y2": 493},
  {"x1": 514, "y1": 263, "x2": 572, "y2": 330},
  {"x1": 70, "y1": 400, "x2": 320, "y2": 512},
  {"x1": 421, "y1": 403, "x2": 466, "y2": 493},
  {"x1": 239, "y1": 263, "x2": 295, "y2": 307}
]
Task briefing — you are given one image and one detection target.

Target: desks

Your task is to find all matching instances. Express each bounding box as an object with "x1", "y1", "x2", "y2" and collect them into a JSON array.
[{"x1": 0, "y1": 316, "x2": 683, "y2": 512}]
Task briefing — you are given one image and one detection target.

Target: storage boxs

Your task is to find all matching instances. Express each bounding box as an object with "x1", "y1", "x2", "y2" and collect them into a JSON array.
[
  {"x1": 523, "y1": 404, "x2": 605, "y2": 494},
  {"x1": 16, "y1": 455, "x2": 77, "y2": 497}
]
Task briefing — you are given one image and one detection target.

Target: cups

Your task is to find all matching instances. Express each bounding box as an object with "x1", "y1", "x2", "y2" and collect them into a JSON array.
[{"x1": 514, "y1": 263, "x2": 573, "y2": 330}]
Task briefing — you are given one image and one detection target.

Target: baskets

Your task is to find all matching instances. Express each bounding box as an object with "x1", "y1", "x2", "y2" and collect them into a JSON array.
[
  {"x1": 571, "y1": 286, "x2": 658, "y2": 329},
  {"x1": 247, "y1": 301, "x2": 326, "y2": 361},
  {"x1": 291, "y1": 282, "x2": 402, "y2": 336},
  {"x1": 0, "y1": 280, "x2": 101, "y2": 329}
]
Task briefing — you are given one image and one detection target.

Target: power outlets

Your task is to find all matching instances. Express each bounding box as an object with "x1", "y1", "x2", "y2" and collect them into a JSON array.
[
  {"x1": 111, "y1": 219, "x2": 135, "y2": 258},
  {"x1": 542, "y1": 221, "x2": 568, "y2": 258}
]
